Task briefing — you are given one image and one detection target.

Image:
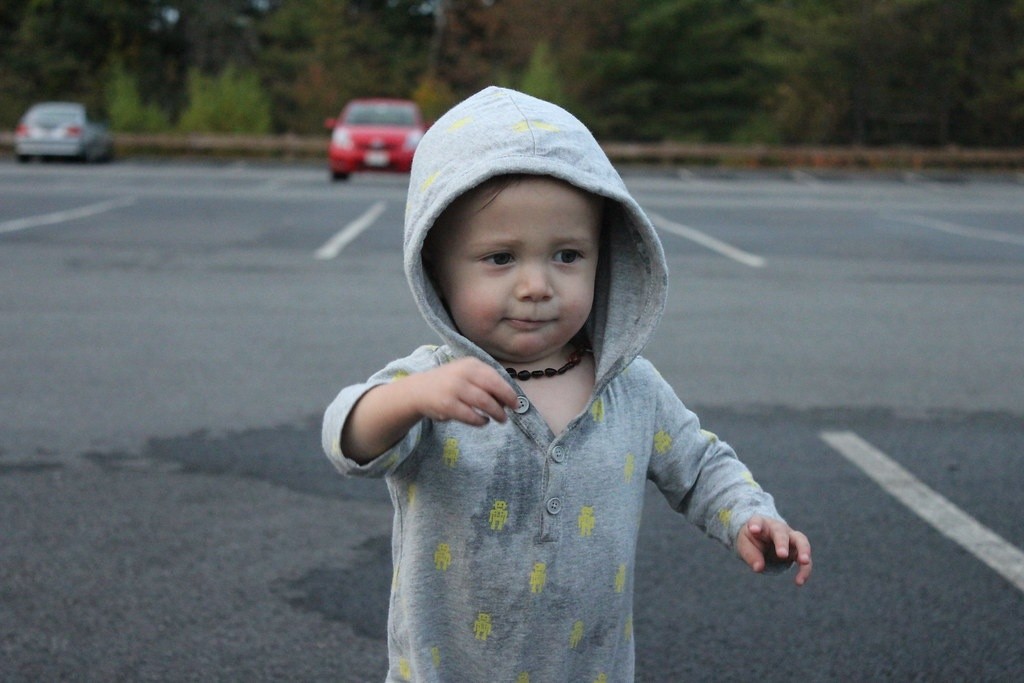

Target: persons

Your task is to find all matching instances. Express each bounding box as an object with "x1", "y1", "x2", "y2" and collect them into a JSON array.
[{"x1": 321, "y1": 85, "x2": 814, "y2": 683}]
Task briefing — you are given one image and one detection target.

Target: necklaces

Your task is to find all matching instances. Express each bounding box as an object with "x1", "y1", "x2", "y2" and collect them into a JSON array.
[{"x1": 505, "y1": 346, "x2": 586, "y2": 381}]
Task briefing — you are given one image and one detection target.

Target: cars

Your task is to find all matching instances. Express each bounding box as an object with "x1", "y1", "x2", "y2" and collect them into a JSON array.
[
  {"x1": 13, "y1": 100, "x2": 117, "y2": 165},
  {"x1": 322, "y1": 96, "x2": 431, "y2": 179}
]
